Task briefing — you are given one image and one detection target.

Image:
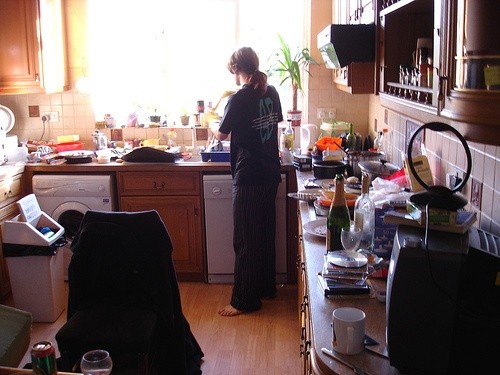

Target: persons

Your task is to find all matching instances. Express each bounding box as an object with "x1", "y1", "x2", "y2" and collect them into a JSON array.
[{"x1": 209, "y1": 46, "x2": 284, "y2": 316}]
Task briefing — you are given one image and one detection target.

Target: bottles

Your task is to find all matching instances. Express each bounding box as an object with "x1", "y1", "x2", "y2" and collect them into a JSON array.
[
  {"x1": 399, "y1": 56, "x2": 432, "y2": 100},
  {"x1": 284, "y1": 119, "x2": 294, "y2": 153},
  {"x1": 347, "y1": 123, "x2": 356, "y2": 151},
  {"x1": 325, "y1": 173, "x2": 351, "y2": 252},
  {"x1": 354, "y1": 174, "x2": 375, "y2": 253}
]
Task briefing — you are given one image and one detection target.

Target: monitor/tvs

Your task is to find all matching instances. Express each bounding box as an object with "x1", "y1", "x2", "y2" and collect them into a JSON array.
[{"x1": 385, "y1": 221, "x2": 500, "y2": 375}]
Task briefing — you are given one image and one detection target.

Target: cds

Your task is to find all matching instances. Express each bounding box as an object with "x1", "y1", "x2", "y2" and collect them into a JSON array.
[{"x1": 327, "y1": 249, "x2": 368, "y2": 268}]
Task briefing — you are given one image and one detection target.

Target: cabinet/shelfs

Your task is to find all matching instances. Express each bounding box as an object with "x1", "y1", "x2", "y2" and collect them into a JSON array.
[
  {"x1": 117, "y1": 166, "x2": 204, "y2": 277},
  {"x1": 295, "y1": 178, "x2": 399, "y2": 375},
  {"x1": 330, "y1": 0, "x2": 376, "y2": 95},
  {"x1": 0, "y1": 0, "x2": 67, "y2": 100},
  {"x1": 374, "y1": 0, "x2": 454, "y2": 120},
  {"x1": 0, "y1": 172, "x2": 30, "y2": 302}
]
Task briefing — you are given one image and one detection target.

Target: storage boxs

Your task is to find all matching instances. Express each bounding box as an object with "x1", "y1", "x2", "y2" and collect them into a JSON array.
[
  {"x1": 57, "y1": 143, "x2": 86, "y2": 153},
  {"x1": 371, "y1": 201, "x2": 405, "y2": 255},
  {"x1": 58, "y1": 134, "x2": 80, "y2": 143},
  {"x1": 200, "y1": 150, "x2": 232, "y2": 162}
]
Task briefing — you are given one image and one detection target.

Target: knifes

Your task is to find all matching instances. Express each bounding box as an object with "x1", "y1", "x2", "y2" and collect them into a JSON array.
[{"x1": 322, "y1": 347, "x2": 369, "y2": 375}]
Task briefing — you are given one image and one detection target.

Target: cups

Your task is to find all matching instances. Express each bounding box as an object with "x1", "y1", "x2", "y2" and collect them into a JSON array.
[
  {"x1": 181, "y1": 116, "x2": 190, "y2": 126},
  {"x1": 376, "y1": 288, "x2": 387, "y2": 302},
  {"x1": 341, "y1": 226, "x2": 362, "y2": 251},
  {"x1": 197, "y1": 100, "x2": 205, "y2": 113},
  {"x1": 81, "y1": 350, "x2": 112, "y2": 375},
  {"x1": 332, "y1": 306, "x2": 367, "y2": 355}
]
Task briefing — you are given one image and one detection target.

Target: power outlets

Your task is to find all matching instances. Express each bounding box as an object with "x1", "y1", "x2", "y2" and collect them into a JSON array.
[
  {"x1": 318, "y1": 108, "x2": 337, "y2": 120},
  {"x1": 41, "y1": 111, "x2": 59, "y2": 122}
]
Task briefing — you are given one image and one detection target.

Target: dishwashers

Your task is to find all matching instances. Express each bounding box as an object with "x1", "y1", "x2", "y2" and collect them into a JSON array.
[{"x1": 202, "y1": 174, "x2": 288, "y2": 285}]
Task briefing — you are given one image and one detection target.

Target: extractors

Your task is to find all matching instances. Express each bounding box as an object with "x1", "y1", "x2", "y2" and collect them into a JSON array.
[{"x1": 317, "y1": 24, "x2": 375, "y2": 70}]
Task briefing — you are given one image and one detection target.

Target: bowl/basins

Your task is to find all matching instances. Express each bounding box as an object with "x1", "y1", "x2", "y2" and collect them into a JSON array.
[
  {"x1": 315, "y1": 136, "x2": 343, "y2": 151},
  {"x1": 313, "y1": 161, "x2": 345, "y2": 179},
  {"x1": 56, "y1": 141, "x2": 94, "y2": 164}
]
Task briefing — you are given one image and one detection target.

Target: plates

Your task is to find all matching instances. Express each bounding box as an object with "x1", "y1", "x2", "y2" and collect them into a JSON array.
[
  {"x1": 319, "y1": 192, "x2": 358, "y2": 206},
  {"x1": 303, "y1": 218, "x2": 328, "y2": 237}
]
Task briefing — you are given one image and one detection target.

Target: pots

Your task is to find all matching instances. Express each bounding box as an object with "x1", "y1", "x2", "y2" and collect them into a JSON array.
[{"x1": 358, "y1": 158, "x2": 402, "y2": 187}]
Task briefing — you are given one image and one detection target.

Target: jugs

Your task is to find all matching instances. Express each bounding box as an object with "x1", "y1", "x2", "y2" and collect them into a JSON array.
[{"x1": 300, "y1": 123, "x2": 320, "y2": 156}]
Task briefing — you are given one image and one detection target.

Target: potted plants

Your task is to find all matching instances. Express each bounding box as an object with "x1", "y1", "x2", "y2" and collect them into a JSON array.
[{"x1": 270, "y1": 35, "x2": 319, "y2": 127}]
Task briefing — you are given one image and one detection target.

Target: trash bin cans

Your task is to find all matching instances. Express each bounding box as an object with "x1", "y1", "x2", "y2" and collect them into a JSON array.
[{"x1": 2, "y1": 195, "x2": 65, "y2": 323}]
